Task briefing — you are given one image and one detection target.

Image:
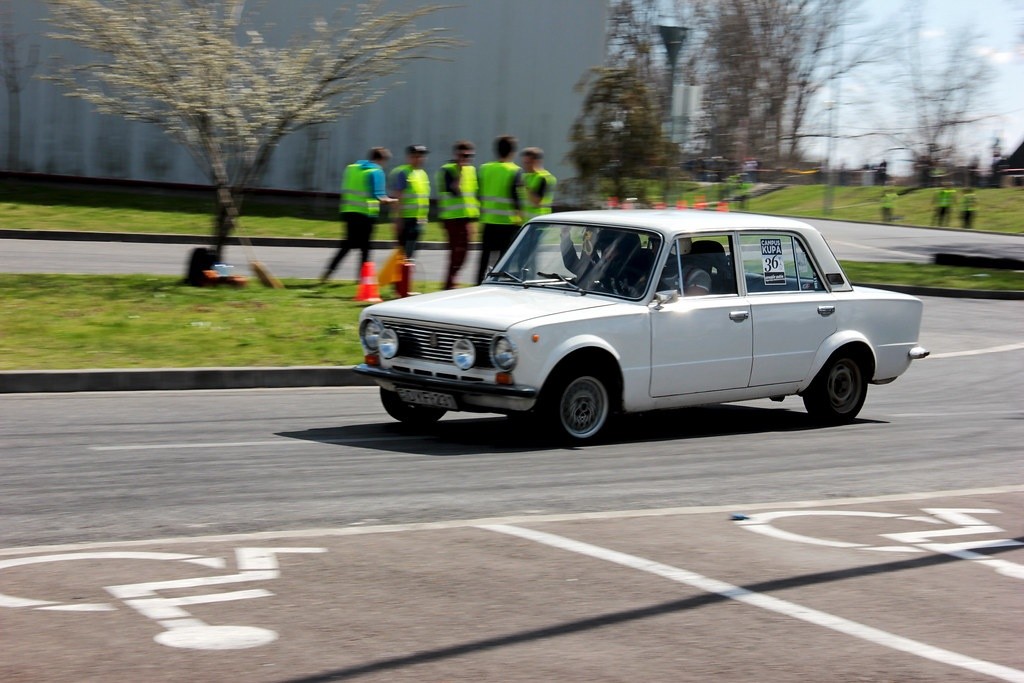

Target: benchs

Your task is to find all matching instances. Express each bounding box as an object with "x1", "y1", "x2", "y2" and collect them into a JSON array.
[{"x1": 746, "y1": 272, "x2": 817, "y2": 292}]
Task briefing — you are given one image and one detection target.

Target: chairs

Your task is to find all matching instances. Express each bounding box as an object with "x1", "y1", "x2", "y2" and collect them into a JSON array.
[{"x1": 692, "y1": 240, "x2": 725, "y2": 293}]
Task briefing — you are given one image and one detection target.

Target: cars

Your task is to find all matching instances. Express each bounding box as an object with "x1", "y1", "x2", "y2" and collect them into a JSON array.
[{"x1": 355, "y1": 207, "x2": 929, "y2": 445}]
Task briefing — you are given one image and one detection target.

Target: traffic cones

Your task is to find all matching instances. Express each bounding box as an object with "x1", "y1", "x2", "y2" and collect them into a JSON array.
[{"x1": 352, "y1": 261, "x2": 387, "y2": 307}]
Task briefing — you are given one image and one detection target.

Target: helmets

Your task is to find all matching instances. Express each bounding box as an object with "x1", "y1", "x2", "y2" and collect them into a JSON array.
[
  {"x1": 593, "y1": 230, "x2": 641, "y2": 271},
  {"x1": 647, "y1": 235, "x2": 694, "y2": 270}
]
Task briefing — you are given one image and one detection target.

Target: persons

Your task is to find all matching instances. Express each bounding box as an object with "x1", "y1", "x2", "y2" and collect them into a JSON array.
[
  {"x1": 321, "y1": 135, "x2": 555, "y2": 294},
  {"x1": 560, "y1": 225, "x2": 712, "y2": 298},
  {"x1": 717, "y1": 160, "x2": 976, "y2": 229}
]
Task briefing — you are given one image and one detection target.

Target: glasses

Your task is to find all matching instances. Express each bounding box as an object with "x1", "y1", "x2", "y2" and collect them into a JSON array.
[{"x1": 460, "y1": 153, "x2": 474, "y2": 159}]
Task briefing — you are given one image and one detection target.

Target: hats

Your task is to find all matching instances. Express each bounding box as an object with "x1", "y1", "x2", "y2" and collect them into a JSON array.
[{"x1": 407, "y1": 144, "x2": 431, "y2": 155}]
